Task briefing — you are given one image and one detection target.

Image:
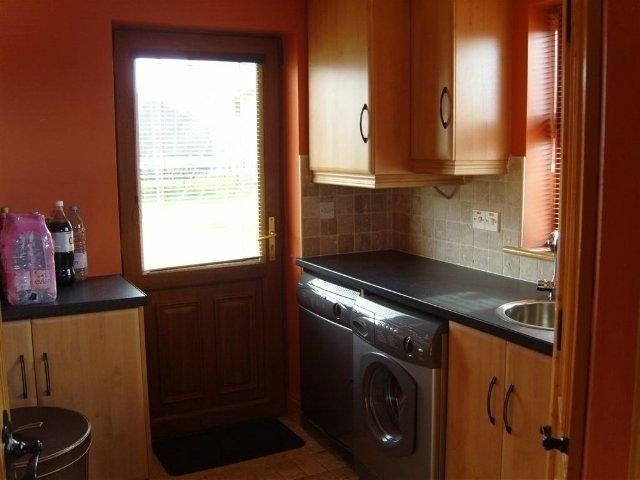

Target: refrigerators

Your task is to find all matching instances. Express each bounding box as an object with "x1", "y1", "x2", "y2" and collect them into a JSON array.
[{"x1": 297, "y1": 269, "x2": 361, "y2": 465}]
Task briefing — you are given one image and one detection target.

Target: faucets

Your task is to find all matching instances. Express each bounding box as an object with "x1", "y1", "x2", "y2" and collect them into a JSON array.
[{"x1": 544, "y1": 232, "x2": 557, "y2": 282}]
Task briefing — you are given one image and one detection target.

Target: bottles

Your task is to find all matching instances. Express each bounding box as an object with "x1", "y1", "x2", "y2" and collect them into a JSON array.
[{"x1": 1, "y1": 201, "x2": 89, "y2": 306}]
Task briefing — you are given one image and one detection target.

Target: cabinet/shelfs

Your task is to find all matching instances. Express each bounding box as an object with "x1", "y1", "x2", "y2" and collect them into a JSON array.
[
  {"x1": 305, "y1": 2, "x2": 513, "y2": 191},
  {"x1": 444, "y1": 320, "x2": 554, "y2": 480},
  {"x1": 2, "y1": 304, "x2": 152, "y2": 478}
]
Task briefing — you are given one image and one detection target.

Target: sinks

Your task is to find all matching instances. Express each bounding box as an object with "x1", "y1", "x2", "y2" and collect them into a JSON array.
[{"x1": 496, "y1": 298, "x2": 555, "y2": 331}]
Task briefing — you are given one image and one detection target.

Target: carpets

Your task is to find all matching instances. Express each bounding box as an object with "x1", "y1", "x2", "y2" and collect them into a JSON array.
[{"x1": 152, "y1": 419, "x2": 303, "y2": 477}]
